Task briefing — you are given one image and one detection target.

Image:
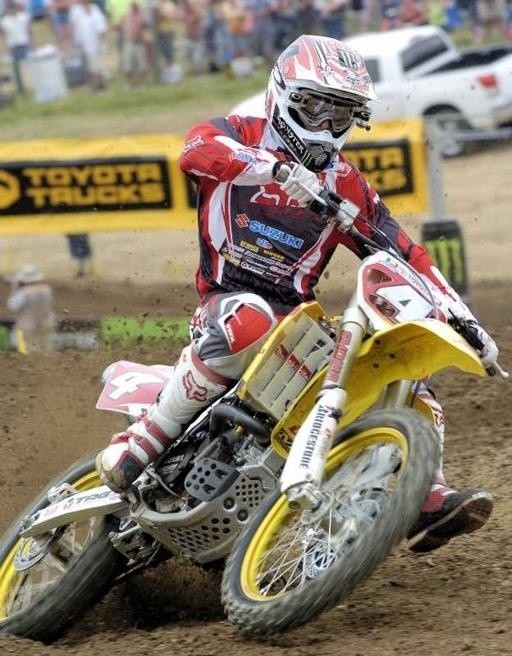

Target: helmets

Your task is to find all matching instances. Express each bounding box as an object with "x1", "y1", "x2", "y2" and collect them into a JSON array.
[{"x1": 264, "y1": 34, "x2": 373, "y2": 173}]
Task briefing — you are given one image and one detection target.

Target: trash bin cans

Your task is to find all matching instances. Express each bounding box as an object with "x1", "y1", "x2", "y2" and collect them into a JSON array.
[{"x1": 18, "y1": 48, "x2": 68, "y2": 102}]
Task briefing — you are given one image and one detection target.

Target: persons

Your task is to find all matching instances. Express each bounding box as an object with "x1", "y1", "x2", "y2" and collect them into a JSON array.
[
  {"x1": 97, "y1": 36, "x2": 499, "y2": 554},
  {"x1": 6, "y1": 258, "x2": 54, "y2": 351}
]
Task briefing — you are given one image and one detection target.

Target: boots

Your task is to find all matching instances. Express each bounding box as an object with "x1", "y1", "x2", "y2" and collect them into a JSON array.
[
  {"x1": 96, "y1": 343, "x2": 239, "y2": 494},
  {"x1": 407, "y1": 397, "x2": 493, "y2": 555}
]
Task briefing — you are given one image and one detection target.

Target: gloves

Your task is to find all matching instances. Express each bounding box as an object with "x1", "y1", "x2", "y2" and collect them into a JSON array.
[
  {"x1": 272, "y1": 160, "x2": 321, "y2": 208},
  {"x1": 466, "y1": 320, "x2": 499, "y2": 369}
]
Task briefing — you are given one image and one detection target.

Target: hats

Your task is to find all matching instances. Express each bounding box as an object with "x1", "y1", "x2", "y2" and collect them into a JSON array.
[{"x1": 17, "y1": 264, "x2": 42, "y2": 283}]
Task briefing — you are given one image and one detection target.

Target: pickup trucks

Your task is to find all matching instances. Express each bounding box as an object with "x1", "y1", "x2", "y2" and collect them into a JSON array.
[{"x1": 224, "y1": 24, "x2": 512, "y2": 157}]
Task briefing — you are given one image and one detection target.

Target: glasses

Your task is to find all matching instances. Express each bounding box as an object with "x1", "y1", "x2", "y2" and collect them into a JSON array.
[{"x1": 301, "y1": 97, "x2": 357, "y2": 132}]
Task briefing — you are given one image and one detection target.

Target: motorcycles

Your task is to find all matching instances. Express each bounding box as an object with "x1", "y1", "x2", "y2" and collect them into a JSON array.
[{"x1": 0, "y1": 165, "x2": 510, "y2": 646}]
